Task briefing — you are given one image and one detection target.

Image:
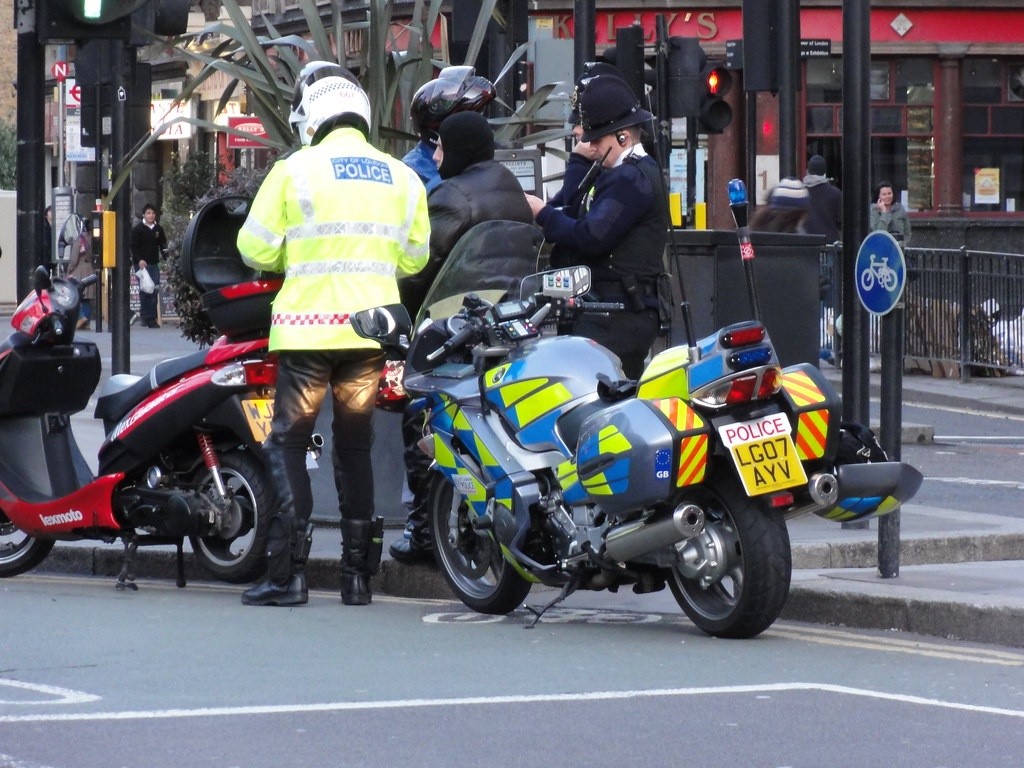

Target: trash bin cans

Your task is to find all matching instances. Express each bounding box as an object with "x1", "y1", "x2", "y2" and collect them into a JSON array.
[{"x1": 668, "y1": 230, "x2": 826, "y2": 370}]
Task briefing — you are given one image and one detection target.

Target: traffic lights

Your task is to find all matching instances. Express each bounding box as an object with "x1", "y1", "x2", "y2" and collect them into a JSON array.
[
  {"x1": 643, "y1": 55, "x2": 660, "y2": 114},
  {"x1": 695, "y1": 59, "x2": 732, "y2": 134}
]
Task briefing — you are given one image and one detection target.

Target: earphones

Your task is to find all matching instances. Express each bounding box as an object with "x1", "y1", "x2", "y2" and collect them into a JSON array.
[{"x1": 617, "y1": 134, "x2": 626, "y2": 144}]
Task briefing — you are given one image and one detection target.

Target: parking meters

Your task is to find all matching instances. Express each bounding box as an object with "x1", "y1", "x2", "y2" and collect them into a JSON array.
[{"x1": 90, "y1": 210, "x2": 116, "y2": 269}]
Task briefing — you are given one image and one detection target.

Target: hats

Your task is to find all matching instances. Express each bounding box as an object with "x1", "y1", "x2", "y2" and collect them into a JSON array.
[
  {"x1": 436, "y1": 112, "x2": 496, "y2": 180},
  {"x1": 808, "y1": 156, "x2": 827, "y2": 175},
  {"x1": 569, "y1": 61, "x2": 657, "y2": 143},
  {"x1": 768, "y1": 176, "x2": 810, "y2": 207}
]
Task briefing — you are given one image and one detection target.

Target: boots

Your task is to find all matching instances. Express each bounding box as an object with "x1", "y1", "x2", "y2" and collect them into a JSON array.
[
  {"x1": 340, "y1": 518, "x2": 375, "y2": 604},
  {"x1": 241, "y1": 518, "x2": 314, "y2": 606}
]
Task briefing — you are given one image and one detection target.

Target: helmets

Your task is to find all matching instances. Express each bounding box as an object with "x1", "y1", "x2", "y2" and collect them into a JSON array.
[
  {"x1": 288, "y1": 61, "x2": 372, "y2": 146},
  {"x1": 410, "y1": 65, "x2": 496, "y2": 148}
]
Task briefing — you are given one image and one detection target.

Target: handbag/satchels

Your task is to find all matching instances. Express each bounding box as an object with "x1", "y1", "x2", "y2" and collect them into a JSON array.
[
  {"x1": 135, "y1": 267, "x2": 155, "y2": 294},
  {"x1": 833, "y1": 422, "x2": 889, "y2": 464},
  {"x1": 78, "y1": 235, "x2": 86, "y2": 254},
  {"x1": 657, "y1": 272, "x2": 674, "y2": 337}
]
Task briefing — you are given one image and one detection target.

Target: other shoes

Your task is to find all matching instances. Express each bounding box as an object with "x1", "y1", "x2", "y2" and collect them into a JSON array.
[
  {"x1": 129, "y1": 311, "x2": 138, "y2": 326},
  {"x1": 140, "y1": 320, "x2": 160, "y2": 328},
  {"x1": 76, "y1": 317, "x2": 91, "y2": 330},
  {"x1": 403, "y1": 527, "x2": 413, "y2": 538}
]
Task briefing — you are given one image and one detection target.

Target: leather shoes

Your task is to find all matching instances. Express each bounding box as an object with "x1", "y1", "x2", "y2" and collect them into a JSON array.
[{"x1": 389, "y1": 538, "x2": 436, "y2": 567}]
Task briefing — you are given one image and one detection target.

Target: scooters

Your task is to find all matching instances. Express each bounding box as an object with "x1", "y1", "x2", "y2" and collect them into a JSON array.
[{"x1": 0, "y1": 196, "x2": 325, "y2": 594}]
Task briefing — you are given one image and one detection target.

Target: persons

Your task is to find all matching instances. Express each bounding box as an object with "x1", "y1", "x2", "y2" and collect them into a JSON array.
[
  {"x1": 67, "y1": 219, "x2": 96, "y2": 330},
  {"x1": 870, "y1": 182, "x2": 912, "y2": 250},
  {"x1": 747, "y1": 155, "x2": 843, "y2": 245},
  {"x1": 236, "y1": 60, "x2": 431, "y2": 606},
  {"x1": 44, "y1": 204, "x2": 57, "y2": 278},
  {"x1": 524, "y1": 62, "x2": 670, "y2": 379},
  {"x1": 388, "y1": 66, "x2": 534, "y2": 570},
  {"x1": 131, "y1": 203, "x2": 169, "y2": 329}
]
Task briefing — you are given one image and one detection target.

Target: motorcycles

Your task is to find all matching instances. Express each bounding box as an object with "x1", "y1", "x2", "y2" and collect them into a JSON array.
[{"x1": 353, "y1": 180, "x2": 923, "y2": 644}]
